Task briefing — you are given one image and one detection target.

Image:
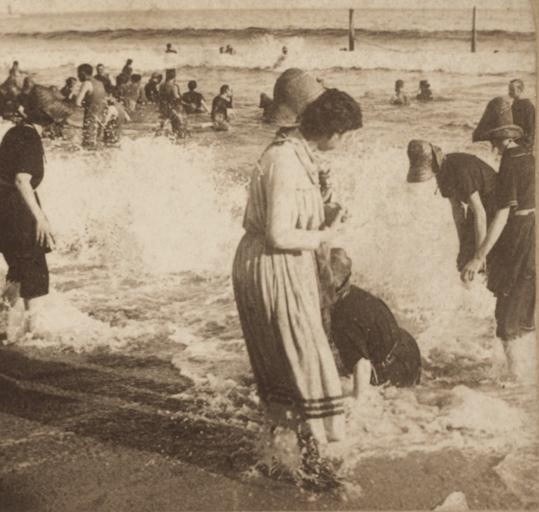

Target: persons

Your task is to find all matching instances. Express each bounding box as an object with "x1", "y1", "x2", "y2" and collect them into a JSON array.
[
  {"x1": 0, "y1": 59, "x2": 234, "y2": 147},
  {"x1": 508, "y1": 79, "x2": 525, "y2": 101},
  {"x1": 165, "y1": 43, "x2": 177, "y2": 54},
  {"x1": 275, "y1": 45, "x2": 291, "y2": 67},
  {"x1": 0, "y1": 88, "x2": 72, "y2": 345},
  {"x1": 471, "y1": 95, "x2": 537, "y2": 384},
  {"x1": 219, "y1": 45, "x2": 236, "y2": 56},
  {"x1": 316, "y1": 244, "x2": 422, "y2": 392},
  {"x1": 391, "y1": 80, "x2": 410, "y2": 105},
  {"x1": 230, "y1": 67, "x2": 363, "y2": 502},
  {"x1": 416, "y1": 80, "x2": 434, "y2": 101},
  {"x1": 402, "y1": 134, "x2": 499, "y2": 274}
]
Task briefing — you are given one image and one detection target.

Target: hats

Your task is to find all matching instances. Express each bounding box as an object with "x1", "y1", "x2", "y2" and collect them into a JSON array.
[
  {"x1": 473, "y1": 96, "x2": 524, "y2": 141},
  {"x1": 262, "y1": 68, "x2": 327, "y2": 128},
  {"x1": 407, "y1": 140, "x2": 444, "y2": 182},
  {"x1": 330, "y1": 248, "x2": 352, "y2": 292},
  {"x1": 23, "y1": 85, "x2": 74, "y2": 125}
]
